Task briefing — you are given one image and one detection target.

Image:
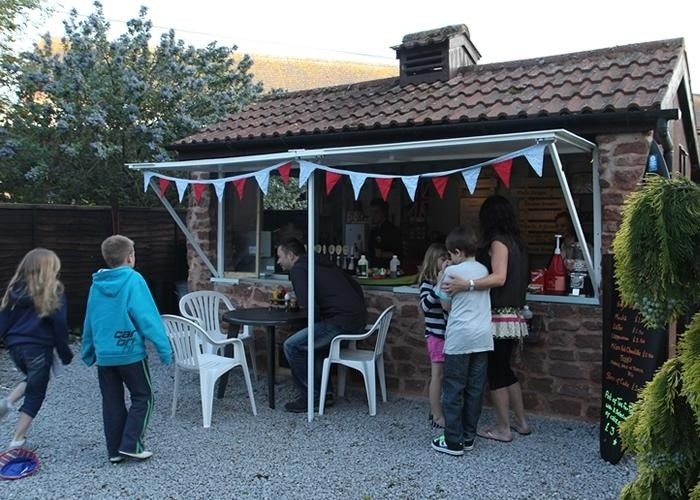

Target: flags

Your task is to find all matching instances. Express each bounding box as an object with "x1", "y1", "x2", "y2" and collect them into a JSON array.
[{"x1": 403, "y1": 184, "x2": 435, "y2": 223}]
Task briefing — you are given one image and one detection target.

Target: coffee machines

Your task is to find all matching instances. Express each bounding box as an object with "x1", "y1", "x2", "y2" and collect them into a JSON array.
[{"x1": 565, "y1": 238, "x2": 593, "y2": 298}]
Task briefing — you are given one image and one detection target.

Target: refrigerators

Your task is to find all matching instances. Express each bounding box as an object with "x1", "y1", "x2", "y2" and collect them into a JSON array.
[{"x1": 344, "y1": 221, "x2": 386, "y2": 258}]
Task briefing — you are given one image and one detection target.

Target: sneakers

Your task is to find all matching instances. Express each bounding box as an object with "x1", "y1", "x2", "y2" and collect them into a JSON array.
[
  {"x1": 284, "y1": 388, "x2": 336, "y2": 413},
  {"x1": 7, "y1": 437, "x2": 38, "y2": 452},
  {"x1": 109, "y1": 448, "x2": 152, "y2": 463},
  {"x1": 0, "y1": 399, "x2": 9, "y2": 418},
  {"x1": 429, "y1": 432, "x2": 475, "y2": 456}
]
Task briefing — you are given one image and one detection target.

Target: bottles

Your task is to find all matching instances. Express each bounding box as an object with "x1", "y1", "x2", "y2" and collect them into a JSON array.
[
  {"x1": 520, "y1": 305, "x2": 533, "y2": 331},
  {"x1": 270, "y1": 285, "x2": 297, "y2": 312},
  {"x1": 390, "y1": 255, "x2": 400, "y2": 277},
  {"x1": 356, "y1": 255, "x2": 369, "y2": 279},
  {"x1": 328, "y1": 256, "x2": 355, "y2": 275}
]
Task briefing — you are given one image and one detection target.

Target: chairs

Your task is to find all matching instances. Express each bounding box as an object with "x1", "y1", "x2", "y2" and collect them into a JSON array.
[
  {"x1": 159, "y1": 314, "x2": 259, "y2": 428},
  {"x1": 180, "y1": 291, "x2": 258, "y2": 386},
  {"x1": 318, "y1": 304, "x2": 395, "y2": 416}
]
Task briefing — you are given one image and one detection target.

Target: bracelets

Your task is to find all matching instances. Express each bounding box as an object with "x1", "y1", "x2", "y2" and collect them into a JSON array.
[{"x1": 470, "y1": 280, "x2": 475, "y2": 293}]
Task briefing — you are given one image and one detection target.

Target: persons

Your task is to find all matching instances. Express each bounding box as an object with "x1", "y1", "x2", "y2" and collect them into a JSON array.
[
  {"x1": 276, "y1": 236, "x2": 368, "y2": 413},
  {"x1": 555, "y1": 210, "x2": 594, "y2": 262},
  {"x1": 417, "y1": 242, "x2": 455, "y2": 430},
  {"x1": 441, "y1": 195, "x2": 531, "y2": 443},
  {"x1": 353, "y1": 198, "x2": 403, "y2": 269},
  {"x1": 429, "y1": 222, "x2": 496, "y2": 456},
  {"x1": 0, "y1": 248, "x2": 73, "y2": 453},
  {"x1": 400, "y1": 239, "x2": 422, "y2": 276},
  {"x1": 80, "y1": 235, "x2": 174, "y2": 463}
]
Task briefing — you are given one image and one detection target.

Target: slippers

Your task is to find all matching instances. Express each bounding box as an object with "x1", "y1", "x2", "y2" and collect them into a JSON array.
[
  {"x1": 476, "y1": 424, "x2": 513, "y2": 443},
  {"x1": 510, "y1": 418, "x2": 532, "y2": 435}
]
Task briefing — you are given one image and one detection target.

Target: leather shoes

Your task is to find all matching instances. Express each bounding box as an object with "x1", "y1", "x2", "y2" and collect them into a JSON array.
[{"x1": 429, "y1": 410, "x2": 446, "y2": 431}]
